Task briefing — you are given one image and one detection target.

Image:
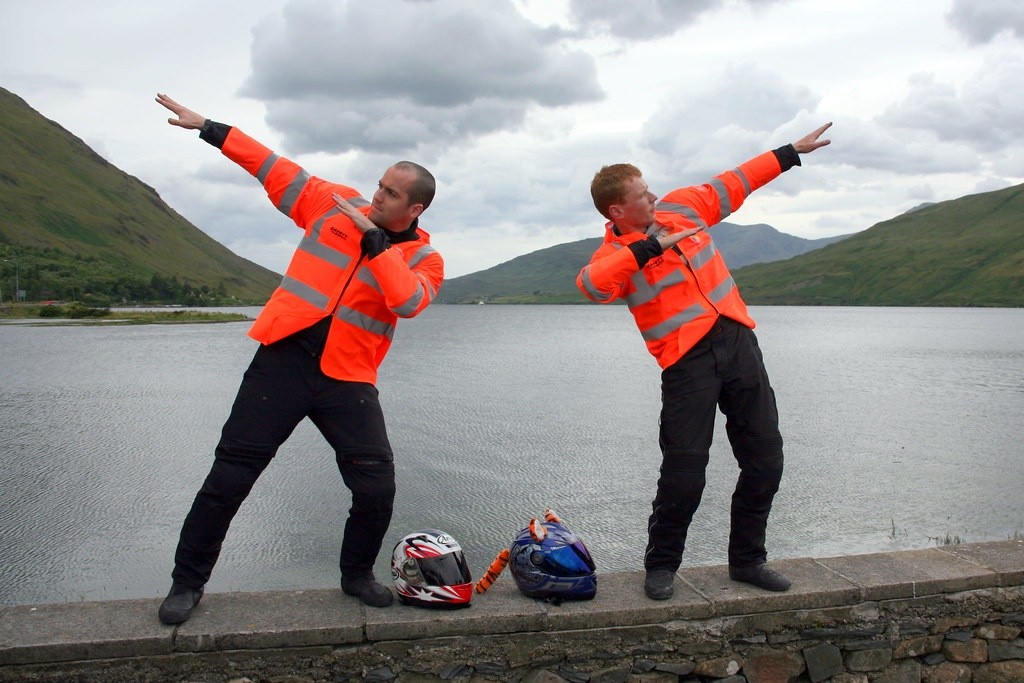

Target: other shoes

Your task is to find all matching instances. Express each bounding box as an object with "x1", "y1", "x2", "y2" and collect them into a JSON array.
[
  {"x1": 158, "y1": 580, "x2": 204, "y2": 625},
  {"x1": 341, "y1": 571, "x2": 394, "y2": 608}
]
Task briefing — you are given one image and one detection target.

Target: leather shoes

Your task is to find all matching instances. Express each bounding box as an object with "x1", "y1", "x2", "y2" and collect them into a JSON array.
[
  {"x1": 645, "y1": 568, "x2": 674, "y2": 600},
  {"x1": 729, "y1": 563, "x2": 791, "y2": 592}
]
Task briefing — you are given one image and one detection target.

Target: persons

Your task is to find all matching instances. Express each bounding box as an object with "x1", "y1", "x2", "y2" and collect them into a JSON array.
[
  {"x1": 575, "y1": 122, "x2": 833, "y2": 601},
  {"x1": 155, "y1": 94, "x2": 444, "y2": 625}
]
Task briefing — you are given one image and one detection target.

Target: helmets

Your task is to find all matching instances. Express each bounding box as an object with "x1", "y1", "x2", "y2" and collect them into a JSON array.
[
  {"x1": 391, "y1": 529, "x2": 472, "y2": 607},
  {"x1": 509, "y1": 522, "x2": 598, "y2": 601}
]
